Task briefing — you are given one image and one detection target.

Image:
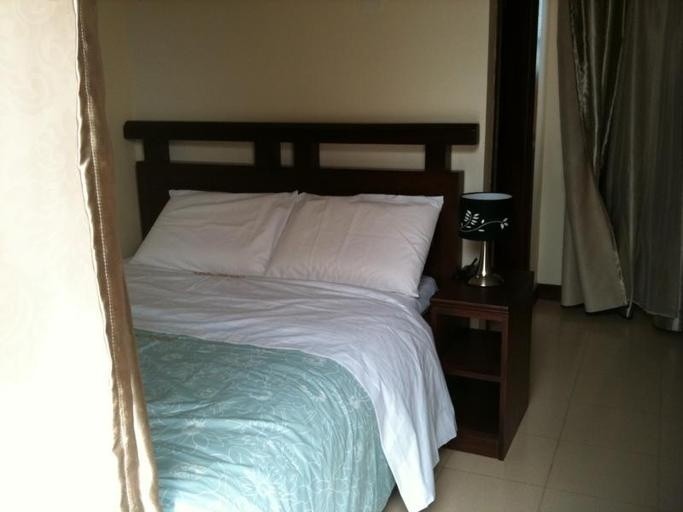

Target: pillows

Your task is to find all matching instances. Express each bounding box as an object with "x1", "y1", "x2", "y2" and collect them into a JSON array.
[
  {"x1": 127, "y1": 188, "x2": 298, "y2": 279},
  {"x1": 267, "y1": 192, "x2": 445, "y2": 299}
]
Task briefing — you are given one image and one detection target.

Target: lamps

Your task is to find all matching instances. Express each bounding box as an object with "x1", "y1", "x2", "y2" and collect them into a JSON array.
[{"x1": 457, "y1": 192, "x2": 522, "y2": 299}]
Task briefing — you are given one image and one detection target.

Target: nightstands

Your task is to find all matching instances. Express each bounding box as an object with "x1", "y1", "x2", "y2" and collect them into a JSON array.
[{"x1": 428, "y1": 271, "x2": 539, "y2": 461}]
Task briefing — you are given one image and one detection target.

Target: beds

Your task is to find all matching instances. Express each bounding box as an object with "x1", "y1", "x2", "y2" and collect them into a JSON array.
[{"x1": 121, "y1": 120, "x2": 477, "y2": 512}]
